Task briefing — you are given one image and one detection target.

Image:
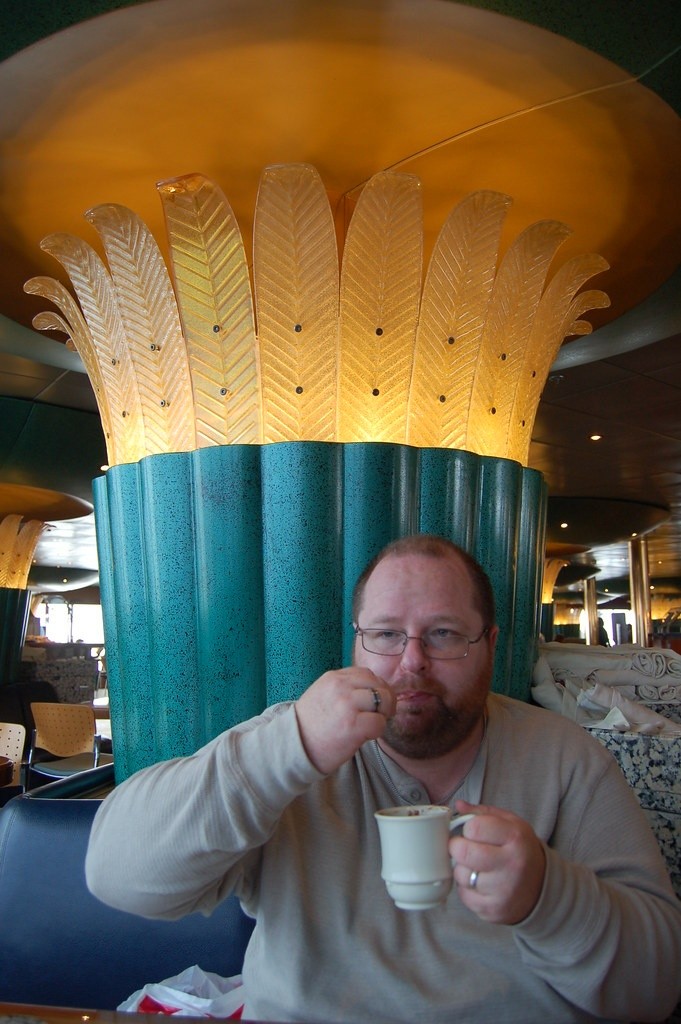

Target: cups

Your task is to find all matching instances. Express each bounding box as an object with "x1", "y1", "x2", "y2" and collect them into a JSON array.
[{"x1": 374, "y1": 804, "x2": 475, "y2": 912}]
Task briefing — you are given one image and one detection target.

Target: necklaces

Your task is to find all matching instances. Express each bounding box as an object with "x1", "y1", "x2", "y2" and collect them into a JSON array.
[{"x1": 372, "y1": 711, "x2": 487, "y2": 806}]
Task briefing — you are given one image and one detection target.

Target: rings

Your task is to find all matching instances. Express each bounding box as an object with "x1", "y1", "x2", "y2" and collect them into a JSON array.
[
  {"x1": 470, "y1": 871, "x2": 478, "y2": 888},
  {"x1": 370, "y1": 687, "x2": 381, "y2": 712}
]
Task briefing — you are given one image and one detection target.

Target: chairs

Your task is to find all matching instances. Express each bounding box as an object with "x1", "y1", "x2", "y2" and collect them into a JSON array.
[
  {"x1": 23, "y1": 701, "x2": 115, "y2": 792},
  {"x1": 0, "y1": 721, "x2": 25, "y2": 802}
]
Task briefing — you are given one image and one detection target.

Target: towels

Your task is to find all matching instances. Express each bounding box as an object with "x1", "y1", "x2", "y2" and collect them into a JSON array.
[{"x1": 530, "y1": 640, "x2": 681, "y2": 737}]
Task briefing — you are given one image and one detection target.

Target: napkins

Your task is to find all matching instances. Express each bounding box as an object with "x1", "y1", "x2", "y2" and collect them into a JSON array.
[{"x1": 534, "y1": 640, "x2": 681, "y2": 741}]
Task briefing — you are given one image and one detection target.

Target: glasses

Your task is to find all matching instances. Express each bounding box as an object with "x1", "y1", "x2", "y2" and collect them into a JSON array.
[{"x1": 352, "y1": 619, "x2": 491, "y2": 660}]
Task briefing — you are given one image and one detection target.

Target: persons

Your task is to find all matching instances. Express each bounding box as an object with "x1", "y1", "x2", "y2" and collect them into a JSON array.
[
  {"x1": 0, "y1": 661, "x2": 60, "y2": 760},
  {"x1": 554, "y1": 634, "x2": 564, "y2": 642},
  {"x1": 599, "y1": 618, "x2": 610, "y2": 647},
  {"x1": 84, "y1": 535, "x2": 681, "y2": 1024}
]
